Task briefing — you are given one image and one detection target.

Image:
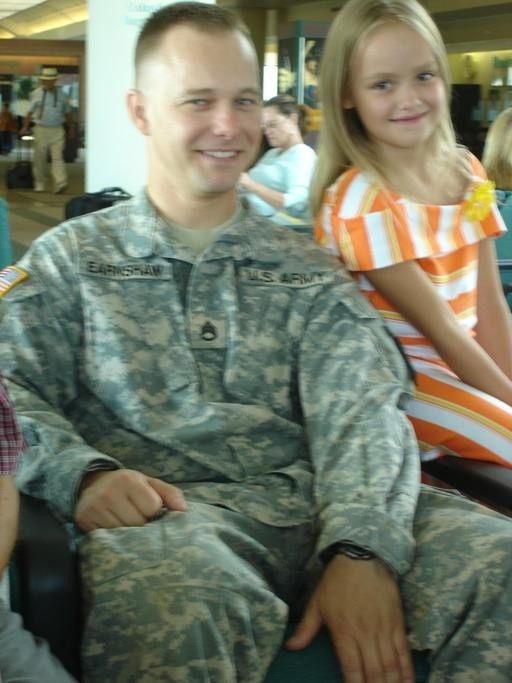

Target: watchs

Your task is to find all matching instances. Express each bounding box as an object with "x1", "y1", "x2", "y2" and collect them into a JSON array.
[{"x1": 321, "y1": 538, "x2": 376, "y2": 567}]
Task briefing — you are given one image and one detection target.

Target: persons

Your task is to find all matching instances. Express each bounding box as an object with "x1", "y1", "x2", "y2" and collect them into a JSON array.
[
  {"x1": 0, "y1": 379, "x2": 79, "y2": 682},
  {"x1": 18, "y1": 66, "x2": 75, "y2": 195},
  {"x1": 0, "y1": 0, "x2": 512, "y2": 683},
  {"x1": 479, "y1": 104, "x2": 512, "y2": 306},
  {"x1": 308, "y1": 0, "x2": 512, "y2": 470},
  {"x1": 233, "y1": 92, "x2": 319, "y2": 229},
  {"x1": 0, "y1": 102, "x2": 18, "y2": 156}
]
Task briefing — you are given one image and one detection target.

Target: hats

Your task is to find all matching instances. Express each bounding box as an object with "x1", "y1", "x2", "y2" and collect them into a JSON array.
[{"x1": 39, "y1": 67, "x2": 62, "y2": 82}]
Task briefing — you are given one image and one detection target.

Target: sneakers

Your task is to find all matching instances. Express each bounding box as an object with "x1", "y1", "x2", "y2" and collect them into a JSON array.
[
  {"x1": 52, "y1": 182, "x2": 70, "y2": 195},
  {"x1": 32, "y1": 181, "x2": 46, "y2": 193}
]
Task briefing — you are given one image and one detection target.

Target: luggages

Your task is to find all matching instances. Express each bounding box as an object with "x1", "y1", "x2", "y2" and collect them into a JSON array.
[
  {"x1": 6, "y1": 132, "x2": 34, "y2": 190},
  {"x1": 64, "y1": 186, "x2": 133, "y2": 221}
]
